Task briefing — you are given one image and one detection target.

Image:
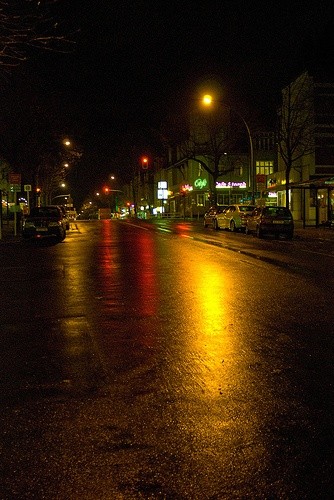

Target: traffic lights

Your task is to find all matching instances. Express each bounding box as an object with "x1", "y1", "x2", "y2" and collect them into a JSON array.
[{"x1": 142, "y1": 156, "x2": 150, "y2": 169}]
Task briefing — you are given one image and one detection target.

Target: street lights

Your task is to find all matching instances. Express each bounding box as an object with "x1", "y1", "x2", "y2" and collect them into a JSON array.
[{"x1": 199, "y1": 89, "x2": 257, "y2": 204}]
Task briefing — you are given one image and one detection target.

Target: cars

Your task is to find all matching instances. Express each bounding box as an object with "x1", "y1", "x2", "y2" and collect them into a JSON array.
[
  {"x1": 22, "y1": 205, "x2": 71, "y2": 239},
  {"x1": 212, "y1": 204, "x2": 257, "y2": 231},
  {"x1": 246, "y1": 206, "x2": 296, "y2": 239},
  {"x1": 203, "y1": 205, "x2": 229, "y2": 229}
]
